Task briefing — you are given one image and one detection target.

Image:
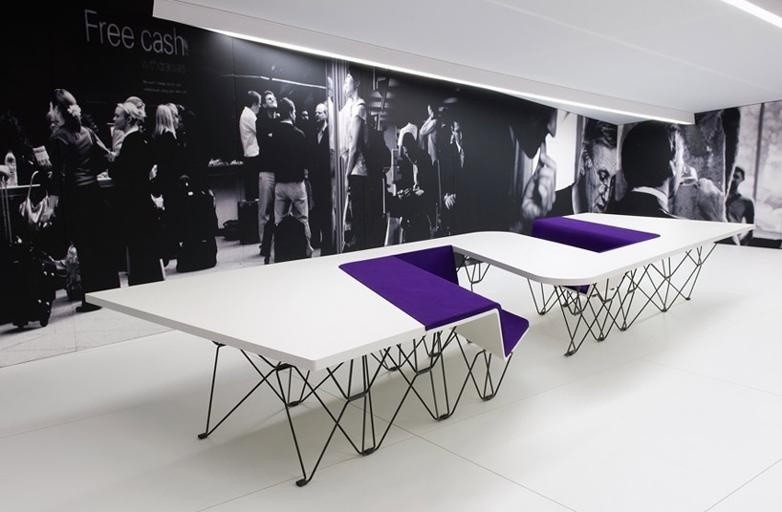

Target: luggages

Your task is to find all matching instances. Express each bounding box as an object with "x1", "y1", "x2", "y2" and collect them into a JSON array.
[
  {"x1": 0, "y1": 176, "x2": 55, "y2": 326},
  {"x1": 237, "y1": 199, "x2": 260, "y2": 244}
]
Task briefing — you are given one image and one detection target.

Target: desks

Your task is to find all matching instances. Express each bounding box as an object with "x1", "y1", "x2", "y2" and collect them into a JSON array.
[{"x1": 83, "y1": 210, "x2": 756, "y2": 490}]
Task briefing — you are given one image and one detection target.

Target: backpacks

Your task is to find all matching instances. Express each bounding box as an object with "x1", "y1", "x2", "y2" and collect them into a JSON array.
[{"x1": 274, "y1": 215, "x2": 307, "y2": 263}]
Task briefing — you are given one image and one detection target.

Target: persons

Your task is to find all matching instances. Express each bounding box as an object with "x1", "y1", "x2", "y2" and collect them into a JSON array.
[
  {"x1": 510, "y1": 97, "x2": 558, "y2": 235},
  {"x1": 1, "y1": 87, "x2": 220, "y2": 331},
  {"x1": 611, "y1": 119, "x2": 724, "y2": 223},
  {"x1": 239, "y1": 66, "x2": 471, "y2": 264},
  {"x1": 723, "y1": 165, "x2": 755, "y2": 244},
  {"x1": 546, "y1": 117, "x2": 619, "y2": 215}
]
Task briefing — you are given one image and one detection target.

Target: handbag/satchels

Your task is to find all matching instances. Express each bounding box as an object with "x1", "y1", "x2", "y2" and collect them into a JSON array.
[{"x1": 19, "y1": 170, "x2": 59, "y2": 231}]
